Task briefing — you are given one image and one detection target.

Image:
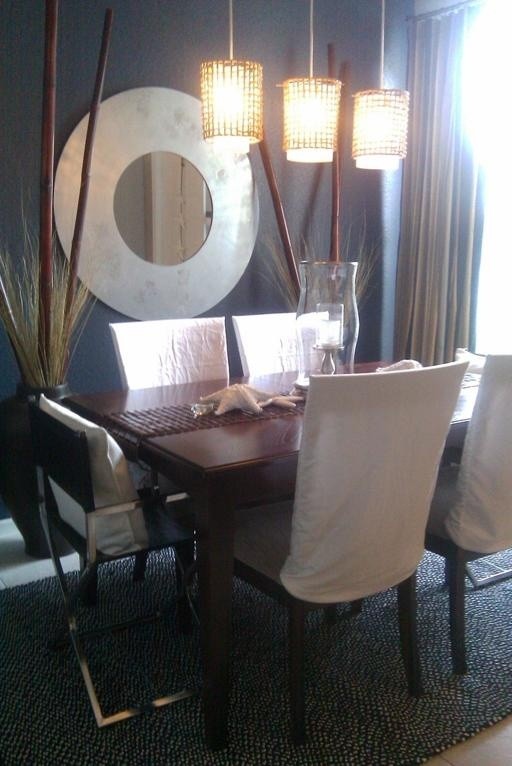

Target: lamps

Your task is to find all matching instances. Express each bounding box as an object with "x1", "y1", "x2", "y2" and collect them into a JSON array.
[{"x1": 200, "y1": 0, "x2": 410, "y2": 170}]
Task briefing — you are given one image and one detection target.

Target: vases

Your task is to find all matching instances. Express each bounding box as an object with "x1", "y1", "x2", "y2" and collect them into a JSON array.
[
  {"x1": 297, "y1": 260, "x2": 360, "y2": 390},
  {"x1": 0, "y1": 381, "x2": 77, "y2": 559}
]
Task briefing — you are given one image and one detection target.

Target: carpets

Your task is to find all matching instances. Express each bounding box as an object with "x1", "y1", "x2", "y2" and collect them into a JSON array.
[{"x1": 0, "y1": 547, "x2": 512, "y2": 766}]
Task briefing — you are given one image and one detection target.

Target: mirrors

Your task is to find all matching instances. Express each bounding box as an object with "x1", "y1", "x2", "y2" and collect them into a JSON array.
[{"x1": 53, "y1": 86, "x2": 260, "y2": 321}]
